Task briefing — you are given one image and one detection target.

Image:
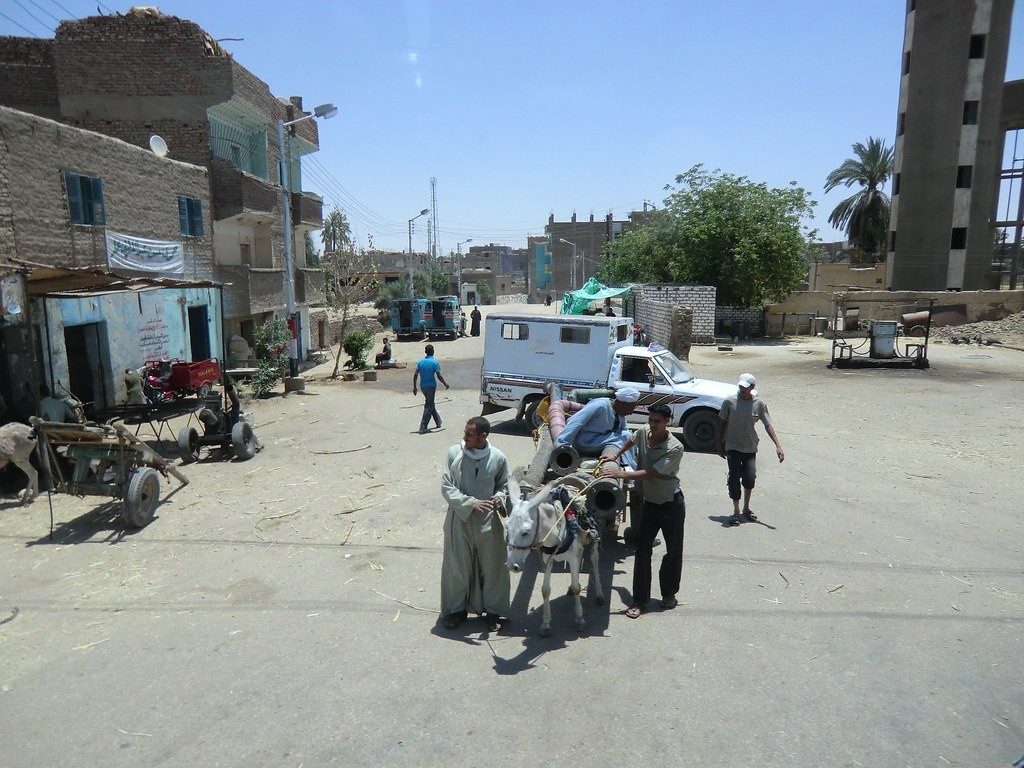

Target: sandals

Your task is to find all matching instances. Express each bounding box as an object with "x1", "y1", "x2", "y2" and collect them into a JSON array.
[
  {"x1": 743, "y1": 509, "x2": 758, "y2": 522},
  {"x1": 660, "y1": 595, "x2": 678, "y2": 609},
  {"x1": 729, "y1": 513, "x2": 741, "y2": 526},
  {"x1": 443, "y1": 610, "x2": 468, "y2": 629},
  {"x1": 625, "y1": 601, "x2": 648, "y2": 618},
  {"x1": 486, "y1": 612, "x2": 502, "y2": 632}
]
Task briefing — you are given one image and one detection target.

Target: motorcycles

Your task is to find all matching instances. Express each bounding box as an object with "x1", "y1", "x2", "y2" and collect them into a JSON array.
[{"x1": 141, "y1": 358, "x2": 220, "y2": 403}]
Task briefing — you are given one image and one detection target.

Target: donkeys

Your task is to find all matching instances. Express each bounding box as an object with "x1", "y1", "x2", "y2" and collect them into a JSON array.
[
  {"x1": 0, "y1": 422, "x2": 38, "y2": 507},
  {"x1": 502, "y1": 475, "x2": 606, "y2": 636}
]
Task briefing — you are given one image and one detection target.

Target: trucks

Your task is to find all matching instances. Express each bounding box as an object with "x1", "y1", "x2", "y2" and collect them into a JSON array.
[
  {"x1": 479, "y1": 312, "x2": 759, "y2": 453},
  {"x1": 424, "y1": 295, "x2": 461, "y2": 339},
  {"x1": 391, "y1": 298, "x2": 430, "y2": 340}
]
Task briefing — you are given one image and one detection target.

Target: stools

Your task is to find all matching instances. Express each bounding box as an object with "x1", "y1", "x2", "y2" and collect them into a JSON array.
[{"x1": 380, "y1": 360, "x2": 389, "y2": 369}]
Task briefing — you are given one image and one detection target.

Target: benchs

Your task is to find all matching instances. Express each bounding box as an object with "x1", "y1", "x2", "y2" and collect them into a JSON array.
[{"x1": 306, "y1": 344, "x2": 336, "y2": 363}]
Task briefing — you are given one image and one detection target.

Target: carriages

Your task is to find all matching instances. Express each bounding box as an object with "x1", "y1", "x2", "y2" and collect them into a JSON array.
[{"x1": 505, "y1": 383, "x2": 630, "y2": 639}]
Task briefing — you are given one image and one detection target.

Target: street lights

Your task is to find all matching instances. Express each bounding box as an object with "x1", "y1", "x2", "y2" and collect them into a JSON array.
[
  {"x1": 409, "y1": 209, "x2": 430, "y2": 298},
  {"x1": 457, "y1": 239, "x2": 473, "y2": 294},
  {"x1": 276, "y1": 103, "x2": 338, "y2": 391},
  {"x1": 559, "y1": 238, "x2": 576, "y2": 290}
]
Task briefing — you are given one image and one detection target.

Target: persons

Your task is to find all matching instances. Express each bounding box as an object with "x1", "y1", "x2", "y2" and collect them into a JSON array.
[
  {"x1": 413, "y1": 345, "x2": 449, "y2": 434},
  {"x1": 375, "y1": 338, "x2": 391, "y2": 369},
  {"x1": 35, "y1": 384, "x2": 77, "y2": 423},
  {"x1": 556, "y1": 386, "x2": 641, "y2": 482},
  {"x1": 606, "y1": 308, "x2": 616, "y2": 316},
  {"x1": 715, "y1": 372, "x2": 784, "y2": 526},
  {"x1": 440, "y1": 416, "x2": 513, "y2": 630},
  {"x1": 125, "y1": 366, "x2": 148, "y2": 405},
  {"x1": 470, "y1": 306, "x2": 481, "y2": 336},
  {"x1": 460, "y1": 313, "x2": 470, "y2": 337},
  {"x1": 599, "y1": 403, "x2": 685, "y2": 618},
  {"x1": 595, "y1": 308, "x2": 606, "y2": 316},
  {"x1": 546, "y1": 294, "x2": 552, "y2": 306},
  {"x1": 583, "y1": 309, "x2": 590, "y2": 315}
]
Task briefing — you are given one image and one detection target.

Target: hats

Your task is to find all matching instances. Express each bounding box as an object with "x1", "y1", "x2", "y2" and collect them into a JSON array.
[
  {"x1": 615, "y1": 387, "x2": 640, "y2": 403},
  {"x1": 737, "y1": 373, "x2": 756, "y2": 388}
]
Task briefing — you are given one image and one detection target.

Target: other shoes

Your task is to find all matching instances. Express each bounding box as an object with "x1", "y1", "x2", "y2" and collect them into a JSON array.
[
  {"x1": 420, "y1": 429, "x2": 433, "y2": 434},
  {"x1": 437, "y1": 420, "x2": 443, "y2": 427}
]
